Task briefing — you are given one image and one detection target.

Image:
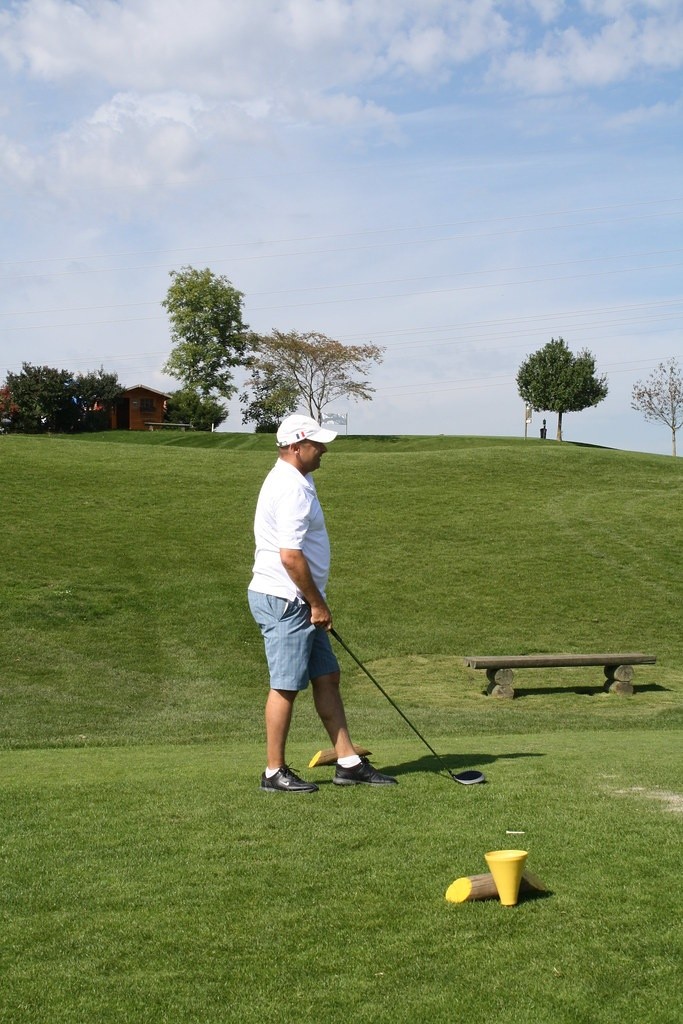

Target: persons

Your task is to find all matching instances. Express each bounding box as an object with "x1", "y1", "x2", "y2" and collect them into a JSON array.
[{"x1": 247, "y1": 415, "x2": 397, "y2": 792}]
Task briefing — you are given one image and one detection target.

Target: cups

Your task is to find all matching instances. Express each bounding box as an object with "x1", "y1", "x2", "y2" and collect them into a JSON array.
[{"x1": 484, "y1": 849, "x2": 528, "y2": 906}]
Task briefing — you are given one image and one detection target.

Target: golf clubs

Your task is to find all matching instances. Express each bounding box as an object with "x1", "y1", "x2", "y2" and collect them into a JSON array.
[{"x1": 301, "y1": 596, "x2": 485, "y2": 785}]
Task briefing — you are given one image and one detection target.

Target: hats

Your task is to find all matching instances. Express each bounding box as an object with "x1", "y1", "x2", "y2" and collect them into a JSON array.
[{"x1": 274, "y1": 414, "x2": 339, "y2": 447}]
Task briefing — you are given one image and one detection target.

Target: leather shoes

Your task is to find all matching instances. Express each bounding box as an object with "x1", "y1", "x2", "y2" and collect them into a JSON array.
[
  {"x1": 332, "y1": 754, "x2": 399, "y2": 786},
  {"x1": 260, "y1": 762, "x2": 319, "y2": 793}
]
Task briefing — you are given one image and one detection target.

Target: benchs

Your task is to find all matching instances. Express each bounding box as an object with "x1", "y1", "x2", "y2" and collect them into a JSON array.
[
  {"x1": 143, "y1": 421, "x2": 190, "y2": 433},
  {"x1": 463, "y1": 652, "x2": 657, "y2": 699}
]
